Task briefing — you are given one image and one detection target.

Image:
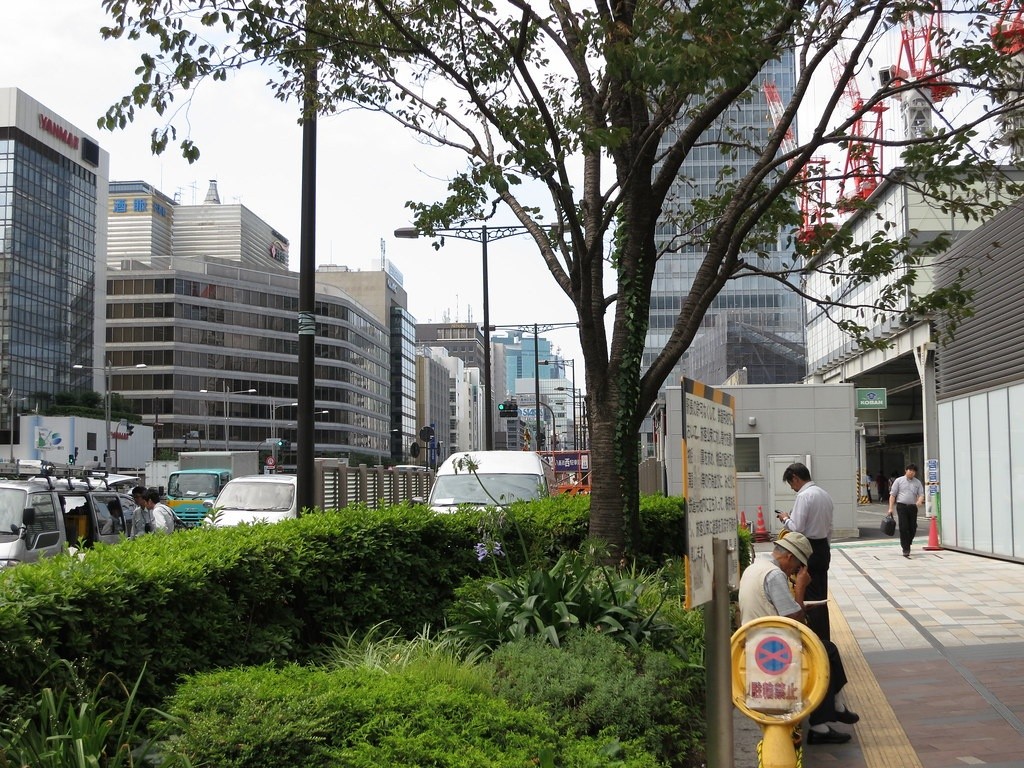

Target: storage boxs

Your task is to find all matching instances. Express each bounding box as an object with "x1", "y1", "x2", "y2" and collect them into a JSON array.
[{"x1": 63, "y1": 513, "x2": 89, "y2": 543}]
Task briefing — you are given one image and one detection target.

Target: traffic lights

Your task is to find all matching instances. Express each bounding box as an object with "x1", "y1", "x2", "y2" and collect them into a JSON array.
[
  {"x1": 498, "y1": 403, "x2": 518, "y2": 417},
  {"x1": 75, "y1": 447, "x2": 78, "y2": 457},
  {"x1": 127, "y1": 425, "x2": 134, "y2": 436},
  {"x1": 279, "y1": 440, "x2": 291, "y2": 448},
  {"x1": 69, "y1": 455, "x2": 73, "y2": 463}
]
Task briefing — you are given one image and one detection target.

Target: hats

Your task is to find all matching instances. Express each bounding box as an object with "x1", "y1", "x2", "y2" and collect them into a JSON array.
[{"x1": 773, "y1": 530, "x2": 815, "y2": 567}]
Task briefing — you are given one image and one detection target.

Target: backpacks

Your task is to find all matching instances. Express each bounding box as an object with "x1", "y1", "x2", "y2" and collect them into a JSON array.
[
  {"x1": 154, "y1": 505, "x2": 189, "y2": 533},
  {"x1": 889, "y1": 478, "x2": 896, "y2": 486}
]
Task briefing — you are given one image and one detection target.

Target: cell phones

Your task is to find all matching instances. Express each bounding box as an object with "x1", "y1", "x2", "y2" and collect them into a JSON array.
[{"x1": 775, "y1": 509, "x2": 781, "y2": 513}]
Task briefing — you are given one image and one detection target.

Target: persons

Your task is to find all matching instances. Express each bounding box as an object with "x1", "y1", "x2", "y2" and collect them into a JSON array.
[
  {"x1": 876, "y1": 471, "x2": 886, "y2": 503},
  {"x1": 101, "y1": 500, "x2": 121, "y2": 533},
  {"x1": 738, "y1": 532, "x2": 859, "y2": 744},
  {"x1": 889, "y1": 472, "x2": 897, "y2": 492},
  {"x1": 866, "y1": 472, "x2": 872, "y2": 503},
  {"x1": 130, "y1": 486, "x2": 152, "y2": 537},
  {"x1": 141, "y1": 487, "x2": 175, "y2": 534},
  {"x1": 889, "y1": 464, "x2": 924, "y2": 556},
  {"x1": 777, "y1": 462, "x2": 835, "y2": 641}
]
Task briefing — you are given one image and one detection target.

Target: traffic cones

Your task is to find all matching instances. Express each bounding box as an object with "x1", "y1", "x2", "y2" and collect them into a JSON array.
[
  {"x1": 741, "y1": 511, "x2": 747, "y2": 529},
  {"x1": 923, "y1": 516, "x2": 943, "y2": 551},
  {"x1": 751, "y1": 506, "x2": 769, "y2": 543}
]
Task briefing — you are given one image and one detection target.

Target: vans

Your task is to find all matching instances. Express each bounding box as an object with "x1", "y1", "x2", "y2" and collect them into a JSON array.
[
  {"x1": 202, "y1": 474, "x2": 300, "y2": 529},
  {"x1": 0, "y1": 460, "x2": 143, "y2": 572},
  {"x1": 413, "y1": 450, "x2": 549, "y2": 516}
]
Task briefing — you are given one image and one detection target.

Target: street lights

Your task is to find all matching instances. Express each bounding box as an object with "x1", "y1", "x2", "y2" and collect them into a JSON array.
[
  {"x1": 200, "y1": 389, "x2": 257, "y2": 451},
  {"x1": 270, "y1": 403, "x2": 398, "y2": 474},
  {"x1": 393, "y1": 222, "x2": 573, "y2": 450},
  {"x1": 538, "y1": 360, "x2": 577, "y2": 451},
  {"x1": 554, "y1": 387, "x2": 585, "y2": 451},
  {"x1": 481, "y1": 321, "x2": 583, "y2": 451},
  {"x1": 73, "y1": 364, "x2": 148, "y2": 472}
]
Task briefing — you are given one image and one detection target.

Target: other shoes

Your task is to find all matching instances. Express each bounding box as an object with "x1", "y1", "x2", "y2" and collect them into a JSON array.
[
  {"x1": 902, "y1": 549, "x2": 910, "y2": 556},
  {"x1": 806, "y1": 725, "x2": 852, "y2": 745},
  {"x1": 808, "y1": 702, "x2": 859, "y2": 726}
]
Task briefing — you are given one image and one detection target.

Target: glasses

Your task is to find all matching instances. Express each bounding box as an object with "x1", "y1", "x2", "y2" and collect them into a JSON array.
[{"x1": 132, "y1": 495, "x2": 143, "y2": 500}]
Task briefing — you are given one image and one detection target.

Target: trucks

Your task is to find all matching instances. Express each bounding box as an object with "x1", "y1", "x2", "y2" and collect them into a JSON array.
[
  {"x1": 145, "y1": 460, "x2": 179, "y2": 503},
  {"x1": 166, "y1": 451, "x2": 259, "y2": 528}
]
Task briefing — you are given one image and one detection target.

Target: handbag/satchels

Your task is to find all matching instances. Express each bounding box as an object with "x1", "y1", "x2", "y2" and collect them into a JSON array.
[{"x1": 880, "y1": 513, "x2": 896, "y2": 536}]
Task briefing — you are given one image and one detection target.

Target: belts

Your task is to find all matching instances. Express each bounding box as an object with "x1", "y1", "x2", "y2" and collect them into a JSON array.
[{"x1": 898, "y1": 502, "x2": 916, "y2": 507}]
